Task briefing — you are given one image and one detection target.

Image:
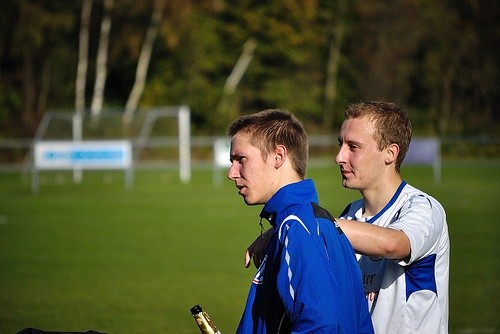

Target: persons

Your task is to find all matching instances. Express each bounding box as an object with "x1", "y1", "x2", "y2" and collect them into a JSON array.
[
  {"x1": 243, "y1": 101, "x2": 451, "y2": 334},
  {"x1": 224, "y1": 109, "x2": 375, "y2": 334}
]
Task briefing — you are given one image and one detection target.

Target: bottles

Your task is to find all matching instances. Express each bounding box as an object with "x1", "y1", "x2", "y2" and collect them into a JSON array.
[{"x1": 190, "y1": 304, "x2": 222, "y2": 334}]
